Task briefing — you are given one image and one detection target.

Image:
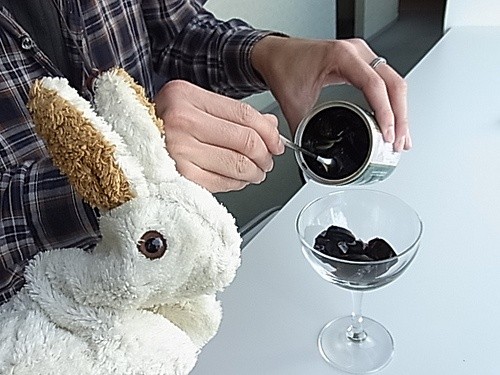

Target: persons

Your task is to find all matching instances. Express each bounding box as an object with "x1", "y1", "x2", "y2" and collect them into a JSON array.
[{"x1": 1, "y1": 1, "x2": 411, "y2": 319}]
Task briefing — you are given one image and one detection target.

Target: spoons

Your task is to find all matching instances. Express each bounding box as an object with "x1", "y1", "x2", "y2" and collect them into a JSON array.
[{"x1": 278, "y1": 133, "x2": 333, "y2": 173}]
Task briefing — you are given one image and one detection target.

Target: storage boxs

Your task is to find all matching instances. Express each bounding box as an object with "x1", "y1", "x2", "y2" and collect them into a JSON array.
[{"x1": 292, "y1": 101, "x2": 401, "y2": 186}]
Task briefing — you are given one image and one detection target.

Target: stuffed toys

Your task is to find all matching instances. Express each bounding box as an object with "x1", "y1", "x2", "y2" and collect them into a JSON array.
[{"x1": 1, "y1": 70, "x2": 242, "y2": 374}]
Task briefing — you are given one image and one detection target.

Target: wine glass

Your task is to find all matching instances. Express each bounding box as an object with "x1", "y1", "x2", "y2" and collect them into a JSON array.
[{"x1": 295, "y1": 188, "x2": 423, "y2": 375}]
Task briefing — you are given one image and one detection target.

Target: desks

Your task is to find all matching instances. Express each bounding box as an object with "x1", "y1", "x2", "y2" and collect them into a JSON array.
[{"x1": 190, "y1": 24, "x2": 500, "y2": 374}]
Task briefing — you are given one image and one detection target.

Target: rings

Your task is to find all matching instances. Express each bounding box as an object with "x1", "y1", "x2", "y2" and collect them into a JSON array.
[{"x1": 369, "y1": 56, "x2": 389, "y2": 69}]
